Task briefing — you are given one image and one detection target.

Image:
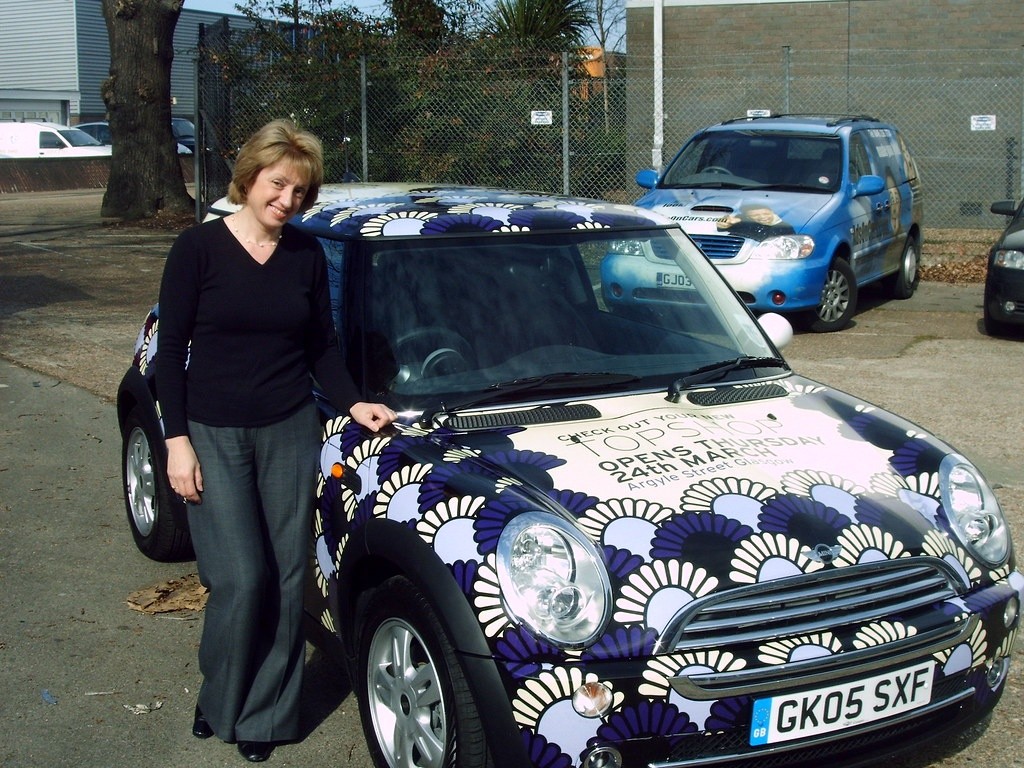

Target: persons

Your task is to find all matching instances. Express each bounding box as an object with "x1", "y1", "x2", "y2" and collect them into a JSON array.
[
  {"x1": 157, "y1": 121, "x2": 398, "y2": 762},
  {"x1": 717, "y1": 204, "x2": 796, "y2": 241}
]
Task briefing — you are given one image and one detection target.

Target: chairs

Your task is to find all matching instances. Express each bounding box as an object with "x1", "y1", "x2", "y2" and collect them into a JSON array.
[
  {"x1": 802, "y1": 149, "x2": 857, "y2": 191},
  {"x1": 471, "y1": 261, "x2": 601, "y2": 376}
]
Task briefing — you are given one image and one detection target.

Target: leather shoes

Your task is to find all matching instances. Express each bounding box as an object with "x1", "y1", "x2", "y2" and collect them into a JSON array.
[
  {"x1": 193, "y1": 703, "x2": 214, "y2": 737},
  {"x1": 237, "y1": 741, "x2": 277, "y2": 762}
]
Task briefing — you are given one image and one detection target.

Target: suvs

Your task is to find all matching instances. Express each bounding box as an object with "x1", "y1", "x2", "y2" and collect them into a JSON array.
[{"x1": 600, "y1": 112, "x2": 926, "y2": 334}]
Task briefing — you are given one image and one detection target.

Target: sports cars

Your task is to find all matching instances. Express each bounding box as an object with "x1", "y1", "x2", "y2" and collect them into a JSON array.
[{"x1": 116, "y1": 182, "x2": 1024, "y2": 768}]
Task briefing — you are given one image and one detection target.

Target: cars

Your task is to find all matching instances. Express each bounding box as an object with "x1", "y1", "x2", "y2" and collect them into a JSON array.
[
  {"x1": 981, "y1": 194, "x2": 1024, "y2": 335},
  {"x1": 171, "y1": 118, "x2": 202, "y2": 152},
  {"x1": 69, "y1": 122, "x2": 193, "y2": 155}
]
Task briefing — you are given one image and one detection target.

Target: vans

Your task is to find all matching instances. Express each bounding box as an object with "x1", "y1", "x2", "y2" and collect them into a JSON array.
[{"x1": 0, "y1": 120, "x2": 115, "y2": 157}]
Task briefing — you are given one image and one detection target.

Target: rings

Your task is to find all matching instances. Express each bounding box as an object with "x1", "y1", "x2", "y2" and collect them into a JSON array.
[{"x1": 171, "y1": 486, "x2": 178, "y2": 489}]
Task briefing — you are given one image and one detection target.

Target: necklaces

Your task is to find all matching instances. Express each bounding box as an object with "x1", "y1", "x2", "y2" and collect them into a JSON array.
[{"x1": 232, "y1": 209, "x2": 282, "y2": 246}]
